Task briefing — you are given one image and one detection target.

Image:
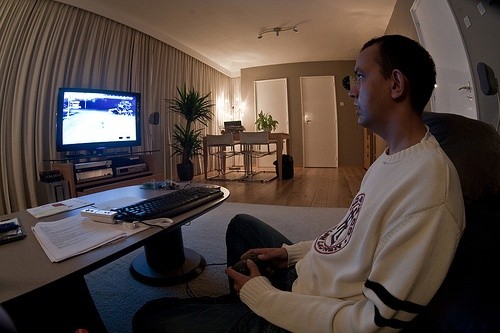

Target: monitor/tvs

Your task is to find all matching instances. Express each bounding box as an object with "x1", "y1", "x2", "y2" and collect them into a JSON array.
[{"x1": 56, "y1": 87, "x2": 141, "y2": 154}]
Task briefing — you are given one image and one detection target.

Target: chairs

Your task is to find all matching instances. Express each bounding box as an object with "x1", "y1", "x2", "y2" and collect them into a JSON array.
[{"x1": 206, "y1": 131, "x2": 285, "y2": 184}]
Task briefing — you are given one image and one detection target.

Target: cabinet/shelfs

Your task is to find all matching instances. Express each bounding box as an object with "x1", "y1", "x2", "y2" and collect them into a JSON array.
[{"x1": 44, "y1": 150, "x2": 159, "y2": 199}]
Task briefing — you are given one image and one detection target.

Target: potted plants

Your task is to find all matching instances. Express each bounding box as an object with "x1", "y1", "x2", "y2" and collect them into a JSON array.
[
  {"x1": 163, "y1": 83, "x2": 216, "y2": 182},
  {"x1": 254, "y1": 110, "x2": 279, "y2": 132}
]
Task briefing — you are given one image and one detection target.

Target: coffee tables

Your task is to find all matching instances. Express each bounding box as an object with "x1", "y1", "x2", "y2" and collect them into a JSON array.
[{"x1": 0, "y1": 182, "x2": 230, "y2": 333}]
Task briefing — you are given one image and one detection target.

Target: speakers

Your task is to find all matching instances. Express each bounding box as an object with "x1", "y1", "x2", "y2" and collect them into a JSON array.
[{"x1": 150, "y1": 112, "x2": 159, "y2": 125}]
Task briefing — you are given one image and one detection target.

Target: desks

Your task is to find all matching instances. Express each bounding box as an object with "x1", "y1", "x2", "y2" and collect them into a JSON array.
[{"x1": 203, "y1": 133, "x2": 291, "y2": 184}]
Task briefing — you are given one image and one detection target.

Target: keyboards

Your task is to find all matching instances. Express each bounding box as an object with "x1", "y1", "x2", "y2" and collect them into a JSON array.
[{"x1": 109, "y1": 185, "x2": 224, "y2": 222}]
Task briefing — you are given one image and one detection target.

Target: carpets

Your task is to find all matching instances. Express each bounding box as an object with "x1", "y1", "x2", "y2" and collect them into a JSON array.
[{"x1": 85, "y1": 201, "x2": 349, "y2": 333}]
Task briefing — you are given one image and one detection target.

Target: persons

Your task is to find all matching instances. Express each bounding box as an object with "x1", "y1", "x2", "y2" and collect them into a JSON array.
[{"x1": 132, "y1": 34, "x2": 466, "y2": 333}]
